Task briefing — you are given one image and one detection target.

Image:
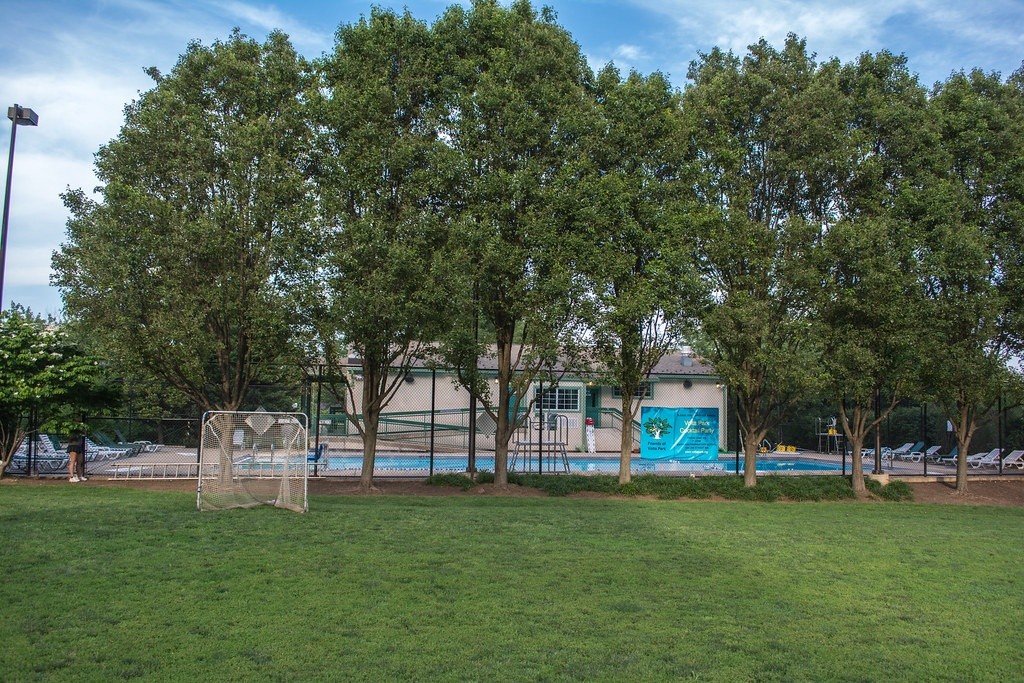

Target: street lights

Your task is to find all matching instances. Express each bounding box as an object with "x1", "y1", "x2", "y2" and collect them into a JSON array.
[{"x1": 0, "y1": 104, "x2": 41, "y2": 310}]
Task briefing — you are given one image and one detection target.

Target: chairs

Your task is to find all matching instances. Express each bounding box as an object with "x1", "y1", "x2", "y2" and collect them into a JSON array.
[
  {"x1": 14, "y1": 430, "x2": 165, "y2": 472},
  {"x1": 306, "y1": 445, "x2": 323, "y2": 475},
  {"x1": 848, "y1": 442, "x2": 1024, "y2": 470}
]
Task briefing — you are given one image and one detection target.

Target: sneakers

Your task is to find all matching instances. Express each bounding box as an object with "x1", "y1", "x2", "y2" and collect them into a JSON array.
[{"x1": 69, "y1": 475, "x2": 87, "y2": 482}]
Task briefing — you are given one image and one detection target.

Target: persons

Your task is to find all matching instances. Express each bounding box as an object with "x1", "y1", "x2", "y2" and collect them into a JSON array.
[{"x1": 65, "y1": 414, "x2": 88, "y2": 483}]
